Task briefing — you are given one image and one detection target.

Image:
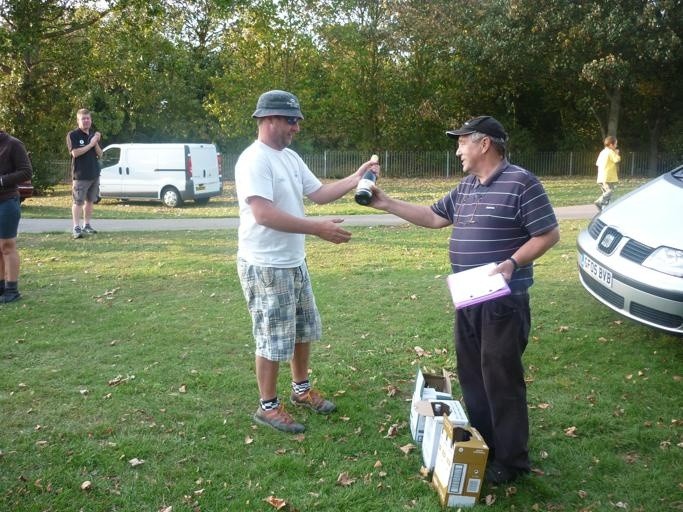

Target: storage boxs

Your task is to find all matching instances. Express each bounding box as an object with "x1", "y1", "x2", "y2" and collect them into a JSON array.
[
  {"x1": 415, "y1": 400, "x2": 467, "y2": 473},
  {"x1": 431, "y1": 413, "x2": 489, "y2": 511},
  {"x1": 409, "y1": 370, "x2": 453, "y2": 444}
]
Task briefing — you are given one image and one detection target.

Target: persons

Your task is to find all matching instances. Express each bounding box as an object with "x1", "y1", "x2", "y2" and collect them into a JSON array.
[
  {"x1": 594, "y1": 136, "x2": 622, "y2": 211},
  {"x1": 365, "y1": 115, "x2": 561, "y2": 486},
  {"x1": 66, "y1": 108, "x2": 103, "y2": 240},
  {"x1": 0, "y1": 130, "x2": 33, "y2": 302},
  {"x1": 234, "y1": 90, "x2": 381, "y2": 434}
]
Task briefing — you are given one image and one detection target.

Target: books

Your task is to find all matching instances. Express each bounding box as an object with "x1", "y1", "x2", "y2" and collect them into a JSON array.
[{"x1": 446, "y1": 261, "x2": 511, "y2": 310}]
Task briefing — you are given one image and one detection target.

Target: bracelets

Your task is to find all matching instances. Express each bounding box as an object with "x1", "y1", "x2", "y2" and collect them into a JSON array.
[{"x1": 506, "y1": 257, "x2": 520, "y2": 272}]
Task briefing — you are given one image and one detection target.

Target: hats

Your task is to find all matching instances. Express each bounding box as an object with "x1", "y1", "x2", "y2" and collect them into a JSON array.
[
  {"x1": 446, "y1": 116, "x2": 507, "y2": 141},
  {"x1": 252, "y1": 89, "x2": 304, "y2": 119}
]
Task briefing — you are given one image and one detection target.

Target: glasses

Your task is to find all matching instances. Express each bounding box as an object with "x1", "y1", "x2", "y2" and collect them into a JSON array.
[{"x1": 283, "y1": 116, "x2": 298, "y2": 125}]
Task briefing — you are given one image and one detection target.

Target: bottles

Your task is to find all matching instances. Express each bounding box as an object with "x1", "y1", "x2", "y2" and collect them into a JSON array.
[
  {"x1": 434, "y1": 404, "x2": 443, "y2": 415},
  {"x1": 462, "y1": 430, "x2": 470, "y2": 441},
  {"x1": 355, "y1": 154, "x2": 380, "y2": 206}
]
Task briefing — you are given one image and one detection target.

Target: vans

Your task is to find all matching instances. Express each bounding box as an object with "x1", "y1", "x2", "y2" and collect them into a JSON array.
[
  {"x1": 95, "y1": 142, "x2": 222, "y2": 208},
  {"x1": 574, "y1": 165, "x2": 683, "y2": 335}
]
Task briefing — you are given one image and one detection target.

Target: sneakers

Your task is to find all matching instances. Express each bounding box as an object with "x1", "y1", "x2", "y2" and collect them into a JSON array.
[
  {"x1": 73, "y1": 226, "x2": 83, "y2": 238},
  {"x1": 595, "y1": 200, "x2": 602, "y2": 210},
  {"x1": 0, "y1": 287, "x2": 20, "y2": 302},
  {"x1": 254, "y1": 403, "x2": 305, "y2": 433},
  {"x1": 81, "y1": 224, "x2": 97, "y2": 234},
  {"x1": 485, "y1": 464, "x2": 521, "y2": 485},
  {"x1": 290, "y1": 388, "x2": 336, "y2": 414}
]
